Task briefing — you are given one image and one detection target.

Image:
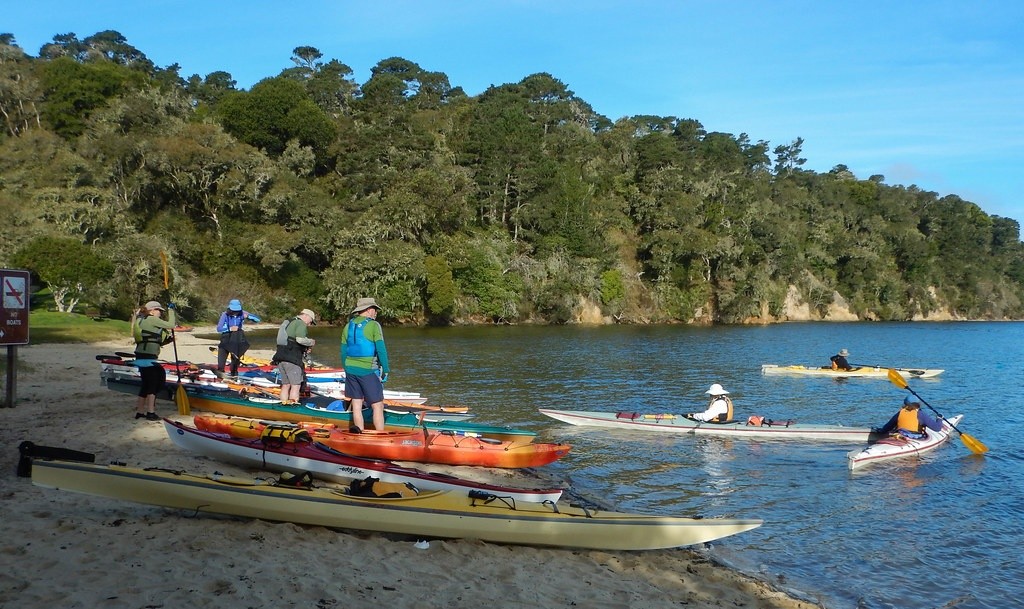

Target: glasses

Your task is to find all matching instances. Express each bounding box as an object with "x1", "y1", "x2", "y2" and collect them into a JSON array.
[
  {"x1": 154, "y1": 309, "x2": 162, "y2": 312},
  {"x1": 370, "y1": 306, "x2": 377, "y2": 312}
]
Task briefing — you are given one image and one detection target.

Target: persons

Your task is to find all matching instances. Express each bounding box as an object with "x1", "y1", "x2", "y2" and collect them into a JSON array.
[
  {"x1": 216, "y1": 300, "x2": 261, "y2": 376},
  {"x1": 341, "y1": 298, "x2": 390, "y2": 431},
  {"x1": 884, "y1": 395, "x2": 944, "y2": 439},
  {"x1": 693, "y1": 384, "x2": 733, "y2": 422},
  {"x1": 135, "y1": 302, "x2": 175, "y2": 421},
  {"x1": 272, "y1": 309, "x2": 316, "y2": 401},
  {"x1": 830, "y1": 349, "x2": 852, "y2": 371}
]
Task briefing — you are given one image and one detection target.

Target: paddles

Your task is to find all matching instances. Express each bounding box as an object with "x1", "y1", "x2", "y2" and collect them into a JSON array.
[
  {"x1": 887, "y1": 369, "x2": 989, "y2": 455},
  {"x1": 160, "y1": 254, "x2": 192, "y2": 416},
  {"x1": 848, "y1": 363, "x2": 925, "y2": 375}
]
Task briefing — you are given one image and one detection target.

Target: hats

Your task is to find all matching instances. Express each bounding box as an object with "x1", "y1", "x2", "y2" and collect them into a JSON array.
[
  {"x1": 145, "y1": 301, "x2": 166, "y2": 311},
  {"x1": 301, "y1": 308, "x2": 317, "y2": 326},
  {"x1": 352, "y1": 298, "x2": 382, "y2": 314},
  {"x1": 838, "y1": 349, "x2": 850, "y2": 356},
  {"x1": 705, "y1": 384, "x2": 729, "y2": 396},
  {"x1": 228, "y1": 299, "x2": 242, "y2": 311}
]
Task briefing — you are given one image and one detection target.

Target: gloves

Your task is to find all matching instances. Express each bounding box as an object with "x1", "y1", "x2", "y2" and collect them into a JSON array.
[
  {"x1": 166, "y1": 303, "x2": 175, "y2": 310},
  {"x1": 381, "y1": 372, "x2": 388, "y2": 383},
  {"x1": 936, "y1": 413, "x2": 944, "y2": 419}
]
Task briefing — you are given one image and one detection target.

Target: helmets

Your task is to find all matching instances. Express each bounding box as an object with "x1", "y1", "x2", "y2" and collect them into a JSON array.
[{"x1": 904, "y1": 393, "x2": 920, "y2": 404}]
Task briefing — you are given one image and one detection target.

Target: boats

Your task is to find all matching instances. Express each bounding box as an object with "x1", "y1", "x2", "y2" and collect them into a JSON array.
[
  {"x1": 193, "y1": 413, "x2": 573, "y2": 468},
  {"x1": 15, "y1": 441, "x2": 766, "y2": 550},
  {"x1": 845, "y1": 413, "x2": 964, "y2": 469},
  {"x1": 537, "y1": 407, "x2": 872, "y2": 441},
  {"x1": 92, "y1": 349, "x2": 480, "y2": 422},
  {"x1": 762, "y1": 364, "x2": 946, "y2": 382},
  {"x1": 173, "y1": 389, "x2": 538, "y2": 443},
  {"x1": 163, "y1": 415, "x2": 563, "y2": 503},
  {"x1": 166, "y1": 324, "x2": 194, "y2": 332}
]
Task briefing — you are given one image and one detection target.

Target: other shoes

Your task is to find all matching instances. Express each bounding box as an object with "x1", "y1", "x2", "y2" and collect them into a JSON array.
[
  {"x1": 146, "y1": 412, "x2": 162, "y2": 420},
  {"x1": 135, "y1": 412, "x2": 146, "y2": 419}
]
